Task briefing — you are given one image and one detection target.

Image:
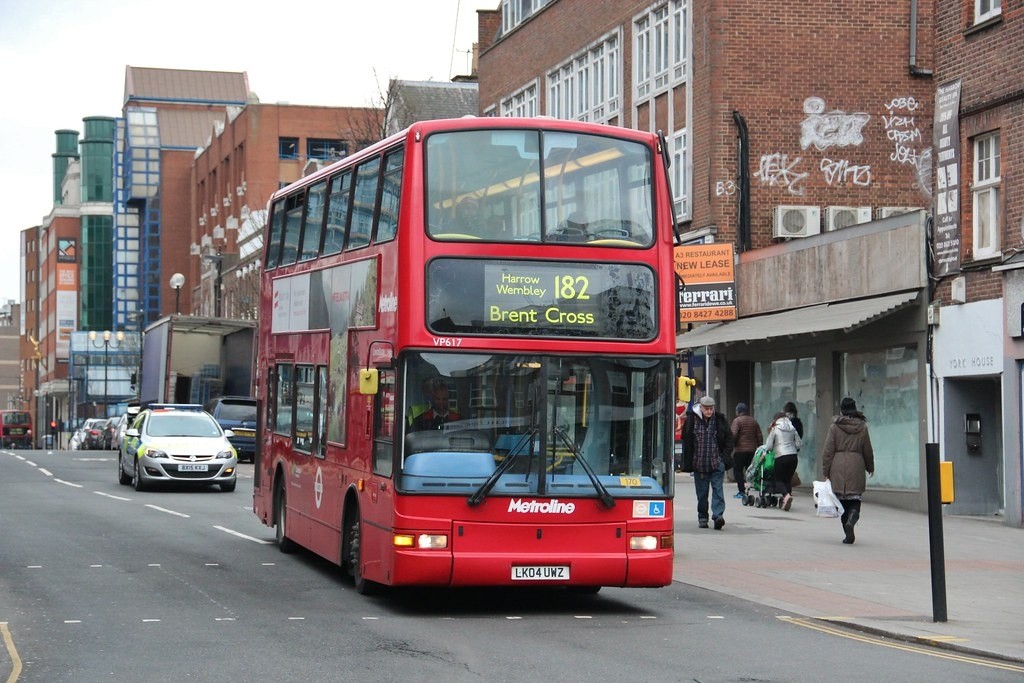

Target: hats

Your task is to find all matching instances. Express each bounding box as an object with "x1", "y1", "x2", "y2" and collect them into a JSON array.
[
  {"x1": 736, "y1": 404, "x2": 748, "y2": 413},
  {"x1": 700, "y1": 396, "x2": 715, "y2": 406}
]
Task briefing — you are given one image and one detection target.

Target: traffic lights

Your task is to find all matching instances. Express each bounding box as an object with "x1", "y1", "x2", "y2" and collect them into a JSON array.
[{"x1": 49, "y1": 420, "x2": 57, "y2": 435}]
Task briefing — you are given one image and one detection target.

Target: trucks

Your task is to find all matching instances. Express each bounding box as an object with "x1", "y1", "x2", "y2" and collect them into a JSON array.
[{"x1": 129, "y1": 312, "x2": 258, "y2": 415}]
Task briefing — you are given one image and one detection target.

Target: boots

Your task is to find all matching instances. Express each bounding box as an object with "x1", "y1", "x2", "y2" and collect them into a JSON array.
[
  {"x1": 843, "y1": 511, "x2": 860, "y2": 543},
  {"x1": 841, "y1": 512, "x2": 852, "y2": 543}
]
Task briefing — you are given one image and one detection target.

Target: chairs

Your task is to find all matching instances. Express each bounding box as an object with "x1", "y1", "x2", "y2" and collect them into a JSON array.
[
  {"x1": 408, "y1": 404, "x2": 460, "y2": 426},
  {"x1": 311, "y1": 218, "x2": 630, "y2": 257}
]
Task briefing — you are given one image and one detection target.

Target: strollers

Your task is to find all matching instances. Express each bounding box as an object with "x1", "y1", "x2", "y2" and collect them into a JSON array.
[{"x1": 740, "y1": 445, "x2": 793, "y2": 509}]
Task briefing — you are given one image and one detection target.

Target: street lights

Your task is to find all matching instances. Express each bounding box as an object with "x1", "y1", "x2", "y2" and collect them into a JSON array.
[
  {"x1": 87, "y1": 329, "x2": 125, "y2": 418},
  {"x1": 168, "y1": 271, "x2": 185, "y2": 314}
]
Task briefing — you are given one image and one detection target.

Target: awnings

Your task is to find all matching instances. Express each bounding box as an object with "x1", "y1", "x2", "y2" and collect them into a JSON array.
[{"x1": 675, "y1": 291, "x2": 920, "y2": 351}]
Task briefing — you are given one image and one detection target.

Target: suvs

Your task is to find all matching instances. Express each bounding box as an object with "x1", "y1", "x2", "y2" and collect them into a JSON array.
[{"x1": 206, "y1": 395, "x2": 257, "y2": 466}]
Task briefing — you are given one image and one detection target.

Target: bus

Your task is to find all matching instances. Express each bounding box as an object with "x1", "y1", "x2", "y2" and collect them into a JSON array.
[
  {"x1": 251, "y1": 111, "x2": 699, "y2": 599},
  {"x1": 0, "y1": 408, "x2": 33, "y2": 448}
]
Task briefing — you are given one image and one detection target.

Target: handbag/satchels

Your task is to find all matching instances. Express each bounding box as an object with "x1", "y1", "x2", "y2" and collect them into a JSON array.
[
  {"x1": 812, "y1": 479, "x2": 844, "y2": 518},
  {"x1": 763, "y1": 449, "x2": 775, "y2": 472},
  {"x1": 725, "y1": 457, "x2": 734, "y2": 470}
]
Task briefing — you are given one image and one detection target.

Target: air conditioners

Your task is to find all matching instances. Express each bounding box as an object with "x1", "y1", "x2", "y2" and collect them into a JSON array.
[
  {"x1": 876, "y1": 207, "x2": 924, "y2": 219},
  {"x1": 773, "y1": 205, "x2": 821, "y2": 238},
  {"x1": 824, "y1": 205, "x2": 872, "y2": 232}
]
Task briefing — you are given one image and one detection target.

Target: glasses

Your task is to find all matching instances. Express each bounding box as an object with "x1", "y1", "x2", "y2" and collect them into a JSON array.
[{"x1": 434, "y1": 397, "x2": 449, "y2": 403}]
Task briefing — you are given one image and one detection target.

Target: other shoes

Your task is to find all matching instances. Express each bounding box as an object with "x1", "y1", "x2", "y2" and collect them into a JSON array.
[
  {"x1": 781, "y1": 493, "x2": 793, "y2": 511},
  {"x1": 734, "y1": 492, "x2": 745, "y2": 497},
  {"x1": 714, "y1": 516, "x2": 725, "y2": 530},
  {"x1": 699, "y1": 521, "x2": 709, "y2": 528}
]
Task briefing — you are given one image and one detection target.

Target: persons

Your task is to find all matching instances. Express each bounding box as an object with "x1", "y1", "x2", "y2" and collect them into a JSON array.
[
  {"x1": 784, "y1": 403, "x2": 804, "y2": 439},
  {"x1": 822, "y1": 398, "x2": 874, "y2": 543},
  {"x1": 776, "y1": 387, "x2": 817, "y2": 474},
  {"x1": 681, "y1": 397, "x2": 733, "y2": 529},
  {"x1": 409, "y1": 383, "x2": 462, "y2": 431},
  {"x1": 556, "y1": 211, "x2": 589, "y2": 242},
  {"x1": 730, "y1": 403, "x2": 763, "y2": 498},
  {"x1": 458, "y1": 197, "x2": 477, "y2": 218},
  {"x1": 4, "y1": 415, "x2": 14, "y2": 424},
  {"x1": 765, "y1": 412, "x2": 802, "y2": 511},
  {"x1": 8, "y1": 442, "x2": 17, "y2": 449},
  {"x1": 24, "y1": 430, "x2": 32, "y2": 448}
]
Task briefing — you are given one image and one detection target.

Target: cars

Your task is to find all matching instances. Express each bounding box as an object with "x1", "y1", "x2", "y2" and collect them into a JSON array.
[
  {"x1": 65, "y1": 406, "x2": 141, "y2": 451},
  {"x1": 118, "y1": 403, "x2": 239, "y2": 492}
]
